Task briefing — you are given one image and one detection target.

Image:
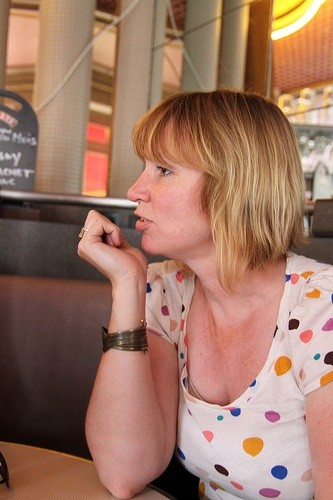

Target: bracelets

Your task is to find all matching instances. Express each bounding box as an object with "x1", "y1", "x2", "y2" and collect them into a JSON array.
[{"x1": 100, "y1": 321, "x2": 149, "y2": 354}]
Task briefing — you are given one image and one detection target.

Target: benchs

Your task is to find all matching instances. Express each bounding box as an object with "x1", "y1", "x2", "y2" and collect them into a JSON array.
[{"x1": 0, "y1": 218, "x2": 333, "y2": 500}]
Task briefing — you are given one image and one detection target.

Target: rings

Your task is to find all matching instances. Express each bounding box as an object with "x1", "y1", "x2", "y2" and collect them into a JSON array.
[{"x1": 79, "y1": 227, "x2": 88, "y2": 238}]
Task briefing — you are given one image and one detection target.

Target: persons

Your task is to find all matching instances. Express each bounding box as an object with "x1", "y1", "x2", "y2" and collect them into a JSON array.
[{"x1": 74, "y1": 88, "x2": 333, "y2": 500}]
